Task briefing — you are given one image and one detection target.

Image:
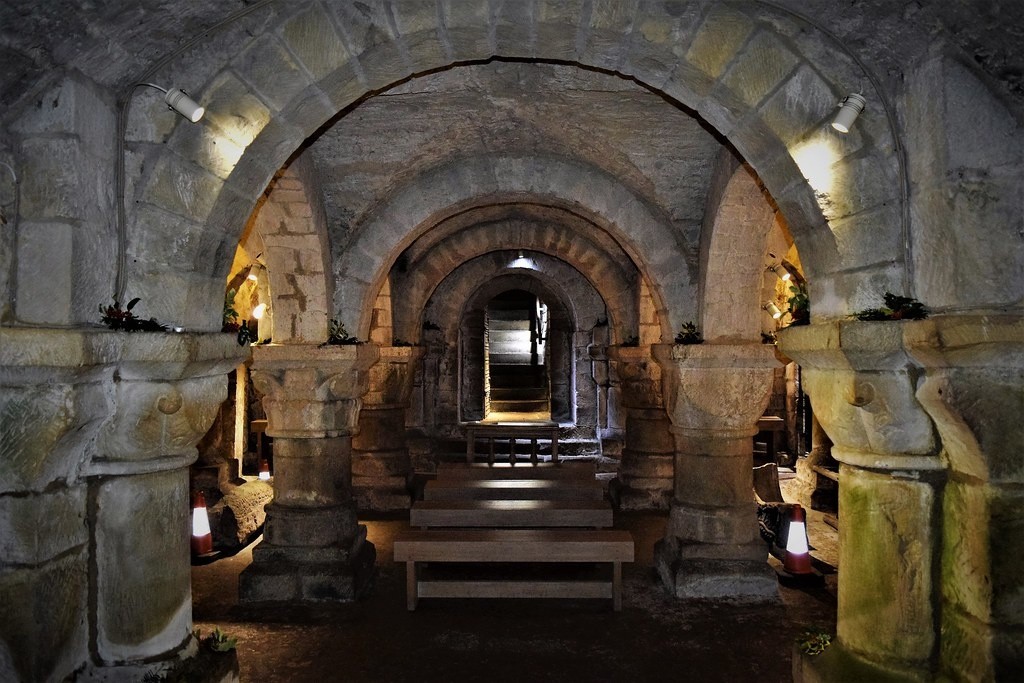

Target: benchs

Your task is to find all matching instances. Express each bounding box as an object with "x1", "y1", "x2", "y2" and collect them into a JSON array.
[
  {"x1": 389, "y1": 462, "x2": 635, "y2": 611},
  {"x1": 753, "y1": 415, "x2": 785, "y2": 464}
]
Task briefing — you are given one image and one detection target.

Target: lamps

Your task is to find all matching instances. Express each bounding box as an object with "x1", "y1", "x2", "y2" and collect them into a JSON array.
[
  {"x1": 770, "y1": 259, "x2": 791, "y2": 281},
  {"x1": 762, "y1": 300, "x2": 781, "y2": 319},
  {"x1": 248, "y1": 263, "x2": 262, "y2": 280},
  {"x1": 164, "y1": 86, "x2": 206, "y2": 124},
  {"x1": 518, "y1": 250, "x2": 523, "y2": 258},
  {"x1": 831, "y1": 80, "x2": 866, "y2": 134},
  {"x1": 764, "y1": 253, "x2": 776, "y2": 271}
]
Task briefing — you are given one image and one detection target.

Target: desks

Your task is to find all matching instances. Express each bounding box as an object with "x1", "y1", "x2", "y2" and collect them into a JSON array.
[{"x1": 459, "y1": 419, "x2": 560, "y2": 465}]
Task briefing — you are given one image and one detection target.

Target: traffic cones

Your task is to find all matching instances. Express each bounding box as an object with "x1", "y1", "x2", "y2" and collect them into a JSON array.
[
  {"x1": 785, "y1": 503, "x2": 815, "y2": 573},
  {"x1": 191, "y1": 490, "x2": 213, "y2": 554},
  {"x1": 256, "y1": 458, "x2": 270, "y2": 481}
]
